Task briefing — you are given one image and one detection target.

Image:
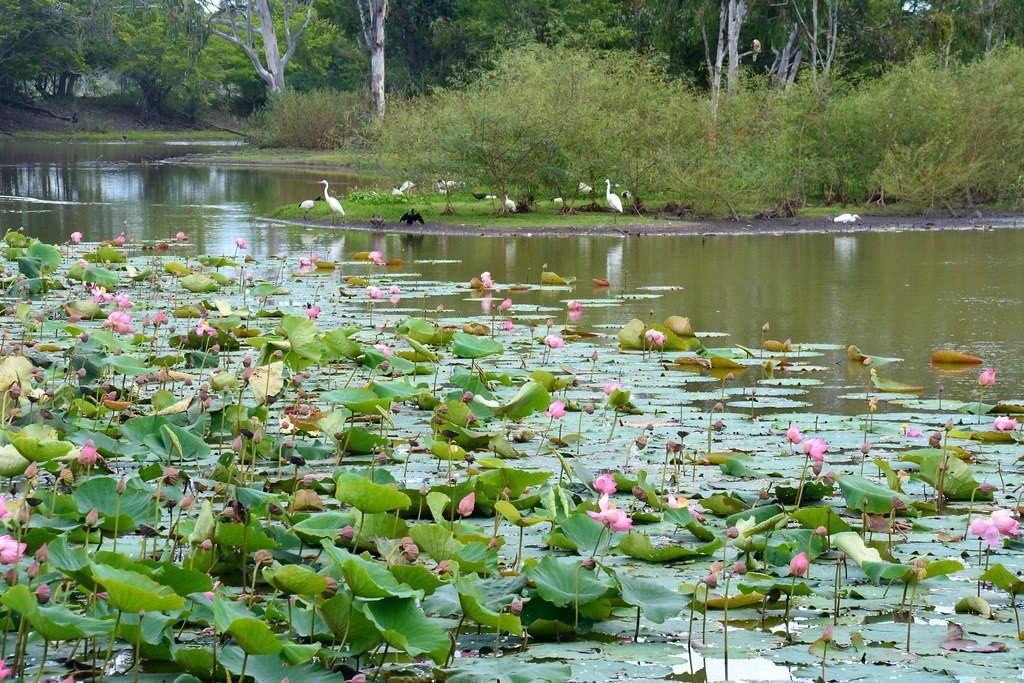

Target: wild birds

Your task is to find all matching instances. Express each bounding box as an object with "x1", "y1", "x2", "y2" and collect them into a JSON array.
[
  {"x1": 299, "y1": 178, "x2": 633, "y2": 228},
  {"x1": 834, "y1": 214, "x2": 860, "y2": 224}
]
